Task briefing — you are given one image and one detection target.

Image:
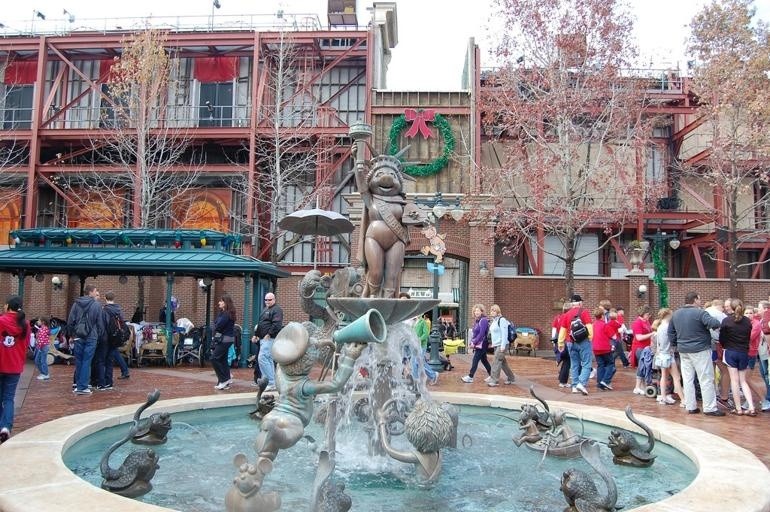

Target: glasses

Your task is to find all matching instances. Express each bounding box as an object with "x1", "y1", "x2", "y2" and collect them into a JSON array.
[{"x1": 264, "y1": 298, "x2": 275, "y2": 302}]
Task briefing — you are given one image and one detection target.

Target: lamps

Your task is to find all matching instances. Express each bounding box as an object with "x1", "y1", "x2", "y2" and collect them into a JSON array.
[
  {"x1": 174, "y1": 236, "x2": 182, "y2": 248},
  {"x1": 198, "y1": 279, "x2": 208, "y2": 293},
  {"x1": 150, "y1": 237, "x2": 156, "y2": 246},
  {"x1": 479, "y1": 260, "x2": 489, "y2": 277},
  {"x1": 39, "y1": 235, "x2": 45, "y2": 244},
  {"x1": 120, "y1": 235, "x2": 129, "y2": 245},
  {"x1": 200, "y1": 235, "x2": 206, "y2": 246},
  {"x1": 65, "y1": 236, "x2": 72, "y2": 244},
  {"x1": 223, "y1": 232, "x2": 234, "y2": 250},
  {"x1": 637, "y1": 285, "x2": 647, "y2": 298},
  {"x1": 52, "y1": 277, "x2": 63, "y2": 291},
  {"x1": 12, "y1": 233, "x2": 20, "y2": 244},
  {"x1": 233, "y1": 233, "x2": 240, "y2": 249}
]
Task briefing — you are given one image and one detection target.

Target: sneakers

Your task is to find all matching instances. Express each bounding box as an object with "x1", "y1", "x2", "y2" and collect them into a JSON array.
[
  {"x1": 215, "y1": 378, "x2": 231, "y2": 389},
  {"x1": 94, "y1": 384, "x2": 114, "y2": 390},
  {"x1": 504, "y1": 380, "x2": 516, "y2": 384},
  {"x1": 633, "y1": 387, "x2": 645, "y2": 395},
  {"x1": 0, "y1": 427, "x2": 10, "y2": 442},
  {"x1": 679, "y1": 401, "x2": 686, "y2": 408},
  {"x1": 559, "y1": 382, "x2": 571, "y2": 388},
  {"x1": 487, "y1": 382, "x2": 500, "y2": 386},
  {"x1": 483, "y1": 375, "x2": 492, "y2": 380},
  {"x1": 461, "y1": 376, "x2": 474, "y2": 384},
  {"x1": 571, "y1": 386, "x2": 581, "y2": 393},
  {"x1": 37, "y1": 373, "x2": 50, "y2": 380},
  {"x1": 429, "y1": 371, "x2": 438, "y2": 385},
  {"x1": 601, "y1": 380, "x2": 614, "y2": 390},
  {"x1": 659, "y1": 399, "x2": 674, "y2": 404},
  {"x1": 576, "y1": 384, "x2": 588, "y2": 394},
  {"x1": 117, "y1": 372, "x2": 131, "y2": 379},
  {"x1": 264, "y1": 384, "x2": 277, "y2": 391},
  {"x1": 73, "y1": 383, "x2": 93, "y2": 396}
]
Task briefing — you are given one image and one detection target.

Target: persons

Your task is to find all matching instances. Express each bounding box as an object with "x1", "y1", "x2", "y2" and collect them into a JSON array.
[
  {"x1": 459, "y1": 303, "x2": 497, "y2": 382},
  {"x1": 397, "y1": 292, "x2": 414, "y2": 300},
  {"x1": 741, "y1": 307, "x2": 770, "y2": 412},
  {"x1": 743, "y1": 305, "x2": 753, "y2": 319},
  {"x1": 653, "y1": 306, "x2": 687, "y2": 407},
  {"x1": 209, "y1": 293, "x2": 235, "y2": 389},
  {"x1": 251, "y1": 292, "x2": 283, "y2": 391},
  {"x1": 95, "y1": 291, "x2": 100, "y2": 300},
  {"x1": 412, "y1": 312, "x2": 438, "y2": 385},
  {"x1": 0, "y1": 295, "x2": 31, "y2": 441},
  {"x1": 67, "y1": 285, "x2": 103, "y2": 394},
  {"x1": 756, "y1": 299, "x2": 770, "y2": 410},
  {"x1": 34, "y1": 315, "x2": 51, "y2": 380},
  {"x1": 551, "y1": 297, "x2": 576, "y2": 387},
  {"x1": 597, "y1": 299, "x2": 613, "y2": 322},
  {"x1": 96, "y1": 291, "x2": 123, "y2": 389},
  {"x1": 592, "y1": 308, "x2": 616, "y2": 391},
  {"x1": 723, "y1": 296, "x2": 734, "y2": 313},
  {"x1": 487, "y1": 304, "x2": 519, "y2": 386},
  {"x1": 719, "y1": 297, "x2": 759, "y2": 416},
  {"x1": 251, "y1": 320, "x2": 262, "y2": 386},
  {"x1": 111, "y1": 346, "x2": 130, "y2": 379},
  {"x1": 631, "y1": 305, "x2": 657, "y2": 395},
  {"x1": 703, "y1": 296, "x2": 737, "y2": 410},
  {"x1": 439, "y1": 321, "x2": 446, "y2": 344},
  {"x1": 422, "y1": 314, "x2": 431, "y2": 350},
  {"x1": 667, "y1": 291, "x2": 725, "y2": 415},
  {"x1": 558, "y1": 294, "x2": 594, "y2": 395},
  {"x1": 615, "y1": 307, "x2": 632, "y2": 369},
  {"x1": 447, "y1": 322, "x2": 455, "y2": 338},
  {"x1": 580, "y1": 359, "x2": 597, "y2": 378}
]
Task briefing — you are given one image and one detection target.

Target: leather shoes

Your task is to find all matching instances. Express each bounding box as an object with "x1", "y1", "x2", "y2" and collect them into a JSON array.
[
  {"x1": 705, "y1": 410, "x2": 726, "y2": 417},
  {"x1": 689, "y1": 408, "x2": 700, "y2": 413}
]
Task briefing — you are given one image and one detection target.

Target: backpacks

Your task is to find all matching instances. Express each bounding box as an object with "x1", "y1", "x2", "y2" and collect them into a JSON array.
[
  {"x1": 570, "y1": 305, "x2": 589, "y2": 342},
  {"x1": 74, "y1": 299, "x2": 93, "y2": 337},
  {"x1": 498, "y1": 315, "x2": 517, "y2": 342},
  {"x1": 104, "y1": 305, "x2": 131, "y2": 346}
]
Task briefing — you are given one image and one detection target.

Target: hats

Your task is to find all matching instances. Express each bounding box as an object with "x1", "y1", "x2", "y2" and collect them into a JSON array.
[{"x1": 570, "y1": 294, "x2": 584, "y2": 302}]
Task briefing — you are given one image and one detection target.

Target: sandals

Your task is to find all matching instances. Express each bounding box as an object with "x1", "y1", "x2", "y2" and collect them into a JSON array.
[
  {"x1": 731, "y1": 409, "x2": 743, "y2": 415},
  {"x1": 742, "y1": 407, "x2": 757, "y2": 418}
]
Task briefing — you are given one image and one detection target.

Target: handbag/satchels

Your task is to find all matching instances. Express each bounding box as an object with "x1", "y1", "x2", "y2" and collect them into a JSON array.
[{"x1": 470, "y1": 338, "x2": 483, "y2": 350}]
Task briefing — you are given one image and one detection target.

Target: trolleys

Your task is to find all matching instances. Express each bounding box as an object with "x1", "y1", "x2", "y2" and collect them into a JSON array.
[
  {"x1": 29, "y1": 317, "x2": 74, "y2": 366},
  {"x1": 135, "y1": 323, "x2": 168, "y2": 367},
  {"x1": 171, "y1": 326, "x2": 206, "y2": 368},
  {"x1": 118, "y1": 323, "x2": 135, "y2": 367}
]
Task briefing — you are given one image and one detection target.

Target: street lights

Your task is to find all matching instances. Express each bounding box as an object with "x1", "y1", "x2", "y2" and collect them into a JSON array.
[
  {"x1": 413, "y1": 191, "x2": 465, "y2": 375},
  {"x1": 638, "y1": 231, "x2": 682, "y2": 308}
]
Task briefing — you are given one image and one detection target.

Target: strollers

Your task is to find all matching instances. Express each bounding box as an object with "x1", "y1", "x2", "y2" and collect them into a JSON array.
[{"x1": 636, "y1": 347, "x2": 663, "y2": 398}]
